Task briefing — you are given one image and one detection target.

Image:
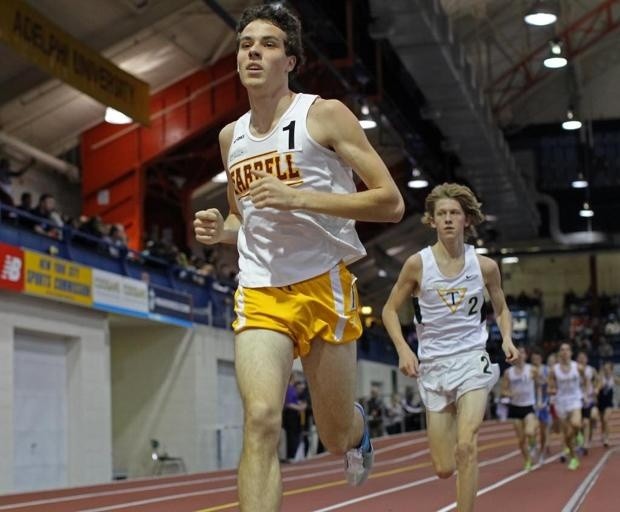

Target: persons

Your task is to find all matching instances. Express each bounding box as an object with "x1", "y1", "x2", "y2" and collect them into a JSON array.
[
  {"x1": 381, "y1": 184, "x2": 520, "y2": 512},
  {"x1": 280, "y1": 373, "x2": 426, "y2": 464},
  {"x1": 193, "y1": 3, "x2": 405, "y2": 512},
  {"x1": 481, "y1": 288, "x2": 620, "y2": 472},
  {"x1": 1, "y1": 182, "x2": 238, "y2": 300}
]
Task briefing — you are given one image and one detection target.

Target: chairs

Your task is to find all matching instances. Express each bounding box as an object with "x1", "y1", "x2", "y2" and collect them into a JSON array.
[{"x1": 148, "y1": 437, "x2": 187, "y2": 477}]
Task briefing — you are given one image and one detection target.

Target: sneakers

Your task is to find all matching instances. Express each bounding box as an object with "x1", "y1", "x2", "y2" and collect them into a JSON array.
[
  {"x1": 517, "y1": 435, "x2": 611, "y2": 474},
  {"x1": 342, "y1": 400, "x2": 375, "y2": 490}
]
